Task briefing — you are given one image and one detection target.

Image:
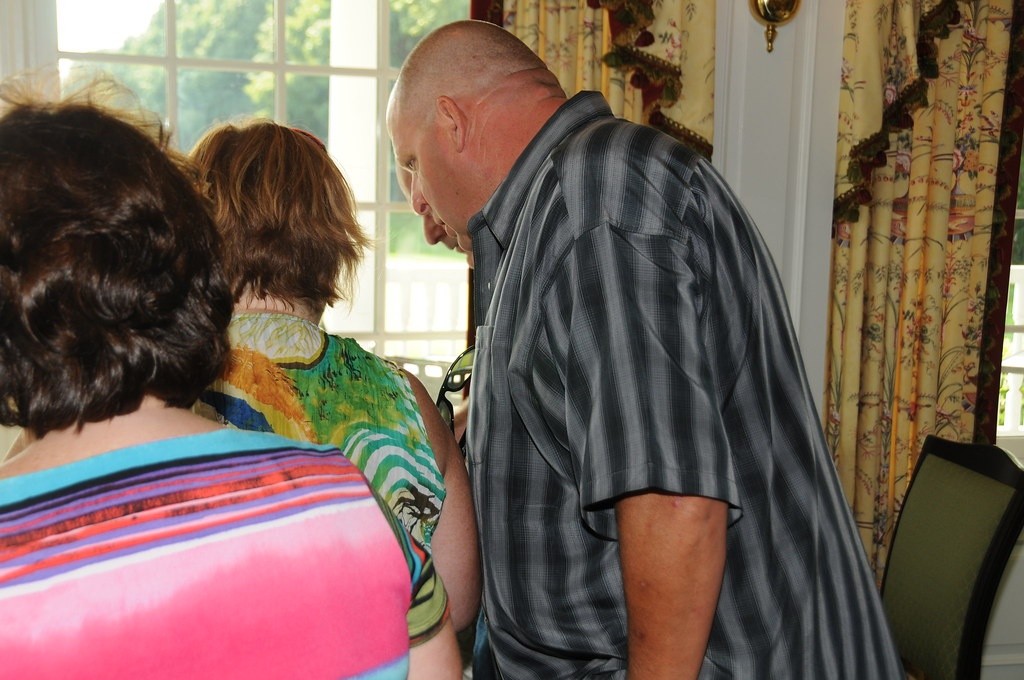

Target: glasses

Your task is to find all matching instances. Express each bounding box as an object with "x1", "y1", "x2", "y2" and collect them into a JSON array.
[{"x1": 435, "y1": 345, "x2": 475, "y2": 437}]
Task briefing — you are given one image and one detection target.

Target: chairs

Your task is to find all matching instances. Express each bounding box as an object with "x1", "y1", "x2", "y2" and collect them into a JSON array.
[{"x1": 880, "y1": 435, "x2": 1024, "y2": 680}]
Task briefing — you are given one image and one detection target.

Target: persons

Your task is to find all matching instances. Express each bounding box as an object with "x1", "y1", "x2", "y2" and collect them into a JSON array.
[
  {"x1": 385, "y1": 20, "x2": 909, "y2": 680},
  {"x1": 1, "y1": 102, "x2": 481, "y2": 680}
]
